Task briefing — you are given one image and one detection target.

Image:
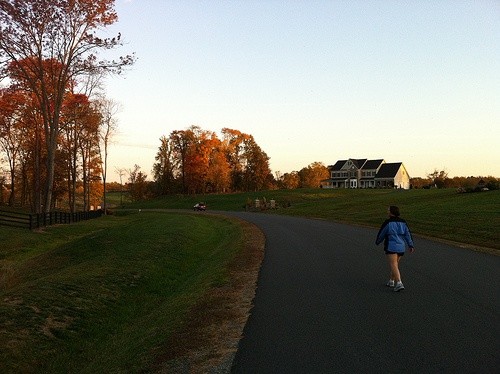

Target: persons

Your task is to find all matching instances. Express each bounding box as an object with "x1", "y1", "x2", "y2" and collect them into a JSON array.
[{"x1": 375, "y1": 206, "x2": 416, "y2": 292}]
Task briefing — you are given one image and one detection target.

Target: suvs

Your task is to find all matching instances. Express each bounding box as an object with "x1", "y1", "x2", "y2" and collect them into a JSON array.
[{"x1": 192, "y1": 202, "x2": 207, "y2": 211}]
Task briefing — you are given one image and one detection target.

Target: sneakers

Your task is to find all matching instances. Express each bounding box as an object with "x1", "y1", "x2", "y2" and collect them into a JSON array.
[
  {"x1": 394, "y1": 283, "x2": 404, "y2": 292},
  {"x1": 387, "y1": 281, "x2": 395, "y2": 287}
]
